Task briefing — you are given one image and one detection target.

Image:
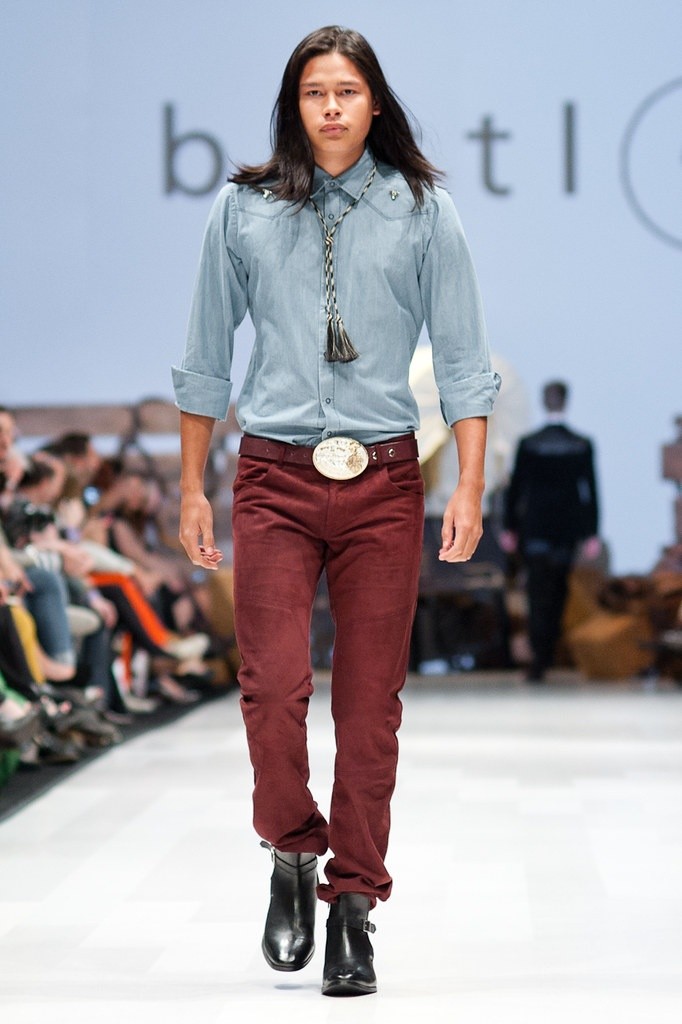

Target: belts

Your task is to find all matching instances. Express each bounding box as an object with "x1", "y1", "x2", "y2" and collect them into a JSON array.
[{"x1": 237, "y1": 431, "x2": 420, "y2": 480}]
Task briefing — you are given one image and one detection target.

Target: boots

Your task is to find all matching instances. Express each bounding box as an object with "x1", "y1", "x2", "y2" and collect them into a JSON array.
[
  {"x1": 258, "y1": 840, "x2": 322, "y2": 973},
  {"x1": 322, "y1": 892, "x2": 378, "y2": 995}
]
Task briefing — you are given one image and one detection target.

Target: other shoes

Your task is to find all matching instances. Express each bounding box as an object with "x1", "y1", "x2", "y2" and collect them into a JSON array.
[{"x1": 1, "y1": 629, "x2": 236, "y2": 771}]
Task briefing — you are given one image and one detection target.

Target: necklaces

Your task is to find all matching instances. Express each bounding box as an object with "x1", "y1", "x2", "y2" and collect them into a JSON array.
[{"x1": 307, "y1": 159, "x2": 380, "y2": 362}]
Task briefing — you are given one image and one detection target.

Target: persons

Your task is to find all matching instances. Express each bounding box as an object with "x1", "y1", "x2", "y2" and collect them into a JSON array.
[
  {"x1": 0, "y1": 405, "x2": 515, "y2": 778},
  {"x1": 171, "y1": 25, "x2": 501, "y2": 995},
  {"x1": 501, "y1": 380, "x2": 600, "y2": 683}
]
